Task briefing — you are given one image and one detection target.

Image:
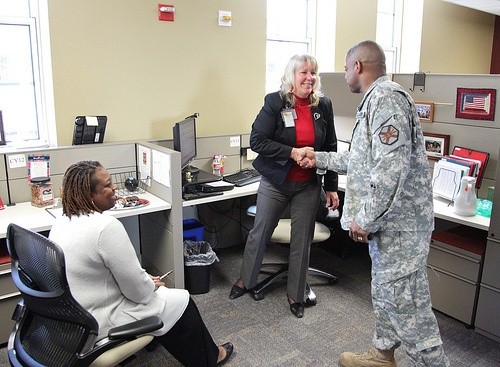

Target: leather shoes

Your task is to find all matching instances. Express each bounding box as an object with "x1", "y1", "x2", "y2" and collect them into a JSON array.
[
  {"x1": 229, "y1": 277, "x2": 247, "y2": 299},
  {"x1": 287, "y1": 292, "x2": 305, "y2": 318},
  {"x1": 210, "y1": 340, "x2": 233, "y2": 367}
]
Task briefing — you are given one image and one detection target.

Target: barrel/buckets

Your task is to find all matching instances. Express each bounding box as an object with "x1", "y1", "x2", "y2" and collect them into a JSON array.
[{"x1": 183, "y1": 218, "x2": 205, "y2": 241}]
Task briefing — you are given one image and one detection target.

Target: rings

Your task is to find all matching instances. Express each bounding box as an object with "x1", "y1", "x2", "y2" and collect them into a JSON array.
[
  {"x1": 306, "y1": 166, "x2": 308, "y2": 168},
  {"x1": 357, "y1": 236, "x2": 363, "y2": 240}
]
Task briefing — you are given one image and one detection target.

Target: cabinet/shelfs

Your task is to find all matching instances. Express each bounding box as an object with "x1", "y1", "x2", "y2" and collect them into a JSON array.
[
  {"x1": 474, "y1": 152, "x2": 500, "y2": 344},
  {"x1": 427, "y1": 217, "x2": 489, "y2": 331}
]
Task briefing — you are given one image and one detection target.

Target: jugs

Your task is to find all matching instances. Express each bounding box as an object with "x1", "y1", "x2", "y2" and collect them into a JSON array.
[{"x1": 454, "y1": 175, "x2": 478, "y2": 217}]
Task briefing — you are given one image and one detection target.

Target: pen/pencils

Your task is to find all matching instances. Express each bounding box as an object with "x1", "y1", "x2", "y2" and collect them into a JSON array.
[
  {"x1": 159, "y1": 270, "x2": 173, "y2": 280},
  {"x1": 214, "y1": 155, "x2": 226, "y2": 164}
]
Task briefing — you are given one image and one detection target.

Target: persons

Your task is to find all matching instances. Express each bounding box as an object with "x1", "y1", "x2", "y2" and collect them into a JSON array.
[
  {"x1": 45, "y1": 160, "x2": 234, "y2": 367},
  {"x1": 297, "y1": 40, "x2": 451, "y2": 367},
  {"x1": 229, "y1": 54, "x2": 339, "y2": 318}
]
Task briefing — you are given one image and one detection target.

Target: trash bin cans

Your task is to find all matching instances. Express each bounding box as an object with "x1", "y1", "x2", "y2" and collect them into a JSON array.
[
  {"x1": 183, "y1": 218, "x2": 204, "y2": 242},
  {"x1": 183, "y1": 241, "x2": 213, "y2": 295}
]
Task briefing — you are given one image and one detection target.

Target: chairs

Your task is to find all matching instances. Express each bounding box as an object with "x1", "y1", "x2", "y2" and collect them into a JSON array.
[
  {"x1": 242, "y1": 200, "x2": 339, "y2": 307},
  {"x1": 6, "y1": 222, "x2": 164, "y2": 367}
]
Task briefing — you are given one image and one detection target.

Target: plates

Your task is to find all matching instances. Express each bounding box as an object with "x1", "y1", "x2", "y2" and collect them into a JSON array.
[{"x1": 109, "y1": 198, "x2": 150, "y2": 210}]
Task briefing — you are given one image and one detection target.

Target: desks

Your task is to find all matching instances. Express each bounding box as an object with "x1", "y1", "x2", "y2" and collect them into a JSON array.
[
  {"x1": 181, "y1": 162, "x2": 492, "y2": 232},
  {"x1": 1, "y1": 188, "x2": 171, "y2": 240}
]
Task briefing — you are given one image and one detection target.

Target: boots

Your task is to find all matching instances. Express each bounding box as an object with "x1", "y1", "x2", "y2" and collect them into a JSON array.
[{"x1": 340, "y1": 346, "x2": 398, "y2": 367}]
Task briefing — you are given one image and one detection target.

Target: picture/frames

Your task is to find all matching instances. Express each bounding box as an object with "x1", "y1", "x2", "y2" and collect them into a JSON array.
[
  {"x1": 422, "y1": 132, "x2": 450, "y2": 162},
  {"x1": 455, "y1": 87, "x2": 495, "y2": 122},
  {"x1": 415, "y1": 102, "x2": 434, "y2": 124}
]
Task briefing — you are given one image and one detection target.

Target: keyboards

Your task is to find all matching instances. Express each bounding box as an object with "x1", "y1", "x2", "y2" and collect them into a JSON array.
[{"x1": 223, "y1": 169, "x2": 262, "y2": 187}]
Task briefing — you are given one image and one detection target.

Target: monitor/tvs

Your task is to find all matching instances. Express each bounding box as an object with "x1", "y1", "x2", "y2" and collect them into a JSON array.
[{"x1": 173, "y1": 117, "x2": 199, "y2": 174}]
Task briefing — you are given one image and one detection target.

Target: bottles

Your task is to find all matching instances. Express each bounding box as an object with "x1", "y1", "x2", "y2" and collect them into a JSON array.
[
  {"x1": 29, "y1": 177, "x2": 55, "y2": 208},
  {"x1": 217, "y1": 154, "x2": 225, "y2": 181},
  {"x1": 211, "y1": 155, "x2": 222, "y2": 175}
]
